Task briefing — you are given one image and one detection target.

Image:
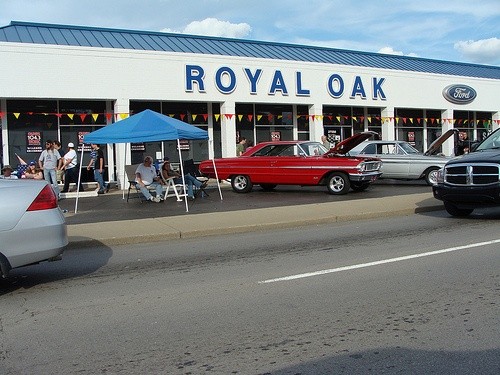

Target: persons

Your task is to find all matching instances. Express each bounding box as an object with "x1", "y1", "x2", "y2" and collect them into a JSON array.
[
  {"x1": 38, "y1": 140, "x2": 62, "y2": 187},
  {"x1": 93, "y1": 144, "x2": 109, "y2": 194},
  {"x1": 0, "y1": 165, "x2": 18, "y2": 180},
  {"x1": 135, "y1": 156, "x2": 164, "y2": 202},
  {"x1": 53, "y1": 141, "x2": 65, "y2": 181},
  {"x1": 237, "y1": 137, "x2": 246, "y2": 156},
  {"x1": 86, "y1": 143, "x2": 96, "y2": 174},
  {"x1": 321, "y1": 136, "x2": 330, "y2": 150},
  {"x1": 60, "y1": 143, "x2": 84, "y2": 192},
  {"x1": 25, "y1": 160, "x2": 45, "y2": 180},
  {"x1": 160, "y1": 157, "x2": 208, "y2": 200}
]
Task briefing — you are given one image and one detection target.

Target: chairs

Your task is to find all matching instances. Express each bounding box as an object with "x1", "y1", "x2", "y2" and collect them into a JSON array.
[{"x1": 125, "y1": 159, "x2": 210, "y2": 204}]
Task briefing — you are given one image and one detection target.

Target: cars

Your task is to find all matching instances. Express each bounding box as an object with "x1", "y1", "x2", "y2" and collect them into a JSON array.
[
  {"x1": 197, "y1": 131, "x2": 383, "y2": 194},
  {"x1": 346, "y1": 128, "x2": 460, "y2": 186},
  {"x1": 0, "y1": 174, "x2": 70, "y2": 285},
  {"x1": 432, "y1": 126, "x2": 500, "y2": 218}
]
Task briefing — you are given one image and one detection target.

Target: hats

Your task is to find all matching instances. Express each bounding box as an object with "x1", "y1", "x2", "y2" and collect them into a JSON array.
[{"x1": 68, "y1": 142, "x2": 74, "y2": 147}]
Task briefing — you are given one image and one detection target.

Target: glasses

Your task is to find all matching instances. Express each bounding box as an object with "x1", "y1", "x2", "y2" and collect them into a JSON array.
[{"x1": 45, "y1": 143, "x2": 50, "y2": 145}]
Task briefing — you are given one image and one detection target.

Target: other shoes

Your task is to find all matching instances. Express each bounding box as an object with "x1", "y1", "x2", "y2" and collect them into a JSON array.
[
  {"x1": 97, "y1": 191, "x2": 104, "y2": 194},
  {"x1": 60, "y1": 190, "x2": 68, "y2": 193},
  {"x1": 200, "y1": 181, "x2": 206, "y2": 189},
  {"x1": 152, "y1": 197, "x2": 160, "y2": 202},
  {"x1": 159, "y1": 195, "x2": 163, "y2": 200},
  {"x1": 106, "y1": 184, "x2": 111, "y2": 193},
  {"x1": 188, "y1": 196, "x2": 195, "y2": 200}
]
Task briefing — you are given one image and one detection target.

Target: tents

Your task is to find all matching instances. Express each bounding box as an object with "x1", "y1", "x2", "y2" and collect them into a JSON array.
[{"x1": 75, "y1": 109, "x2": 223, "y2": 213}]
